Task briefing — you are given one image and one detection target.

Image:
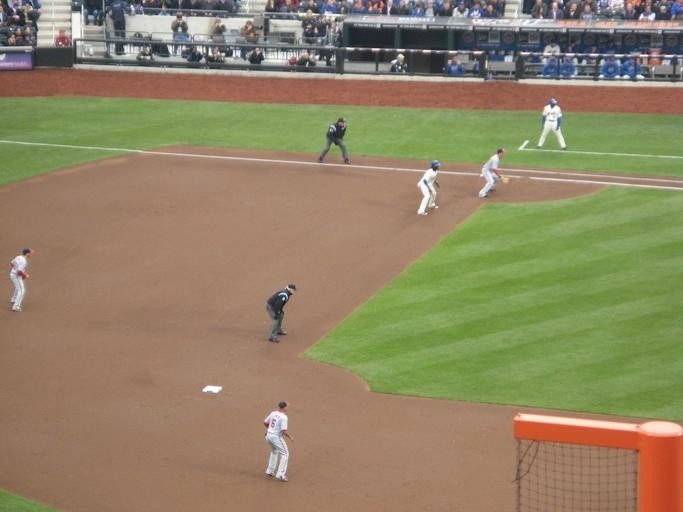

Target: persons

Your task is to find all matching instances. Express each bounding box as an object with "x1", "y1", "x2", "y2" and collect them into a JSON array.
[
  {"x1": 315, "y1": 118, "x2": 351, "y2": 164},
  {"x1": 416, "y1": 159, "x2": 441, "y2": 216},
  {"x1": 536, "y1": 98, "x2": 567, "y2": 151},
  {"x1": 477, "y1": 148, "x2": 509, "y2": 199},
  {"x1": 9, "y1": 248, "x2": 34, "y2": 312},
  {"x1": 263, "y1": 401, "x2": 296, "y2": 481},
  {"x1": 265, "y1": 283, "x2": 298, "y2": 343}
]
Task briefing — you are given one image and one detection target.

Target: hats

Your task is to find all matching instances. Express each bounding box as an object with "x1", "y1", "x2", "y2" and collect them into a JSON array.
[
  {"x1": 278, "y1": 400, "x2": 289, "y2": 408},
  {"x1": 338, "y1": 117, "x2": 346, "y2": 122},
  {"x1": 22, "y1": 248, "x2": 33, "y2": 256},
  {"x1": 287, "y1": 283, "x2": 297, "y2": 291}
]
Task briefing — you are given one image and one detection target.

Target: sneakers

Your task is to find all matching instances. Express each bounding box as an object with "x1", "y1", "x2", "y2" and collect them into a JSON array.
[
  {"x1": 275, "y1": 474, "x2": 289, "y2": 482},
  {"x1": 265, "y1": 471, "x2": 275, "y2": 477},
  {"x1": 9, "y1": 300, "x2": 23, "y2": 312},
  {"x1": 269, "y1": 336, "x2": 279, "y2": 343},
  {"x1": 428, "y1": 204, "x2": 439, "y2": 209},
  {"x1": 417, "y1": 210, "x2": 428, "y2": 216},
  {"x1": 277, "y1": 328, "x2": 287, "y2": 335},
  {"x1": 344, "y1": 160, "x2": 352, "y2": 165}
]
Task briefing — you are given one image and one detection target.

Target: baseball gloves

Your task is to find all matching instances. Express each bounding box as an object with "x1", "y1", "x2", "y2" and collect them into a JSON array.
[{"x1": 500, "y1": 175, "x2": 509, "y2": 185}]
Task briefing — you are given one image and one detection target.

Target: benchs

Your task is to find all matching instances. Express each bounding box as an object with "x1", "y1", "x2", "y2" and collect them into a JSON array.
[{"x1": 522, "y1": 61, "x2": 654, "y2": 82}]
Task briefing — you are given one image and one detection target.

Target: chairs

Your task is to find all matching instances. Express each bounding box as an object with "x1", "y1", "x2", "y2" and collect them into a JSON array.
[{"x1": 129, "y1": 28, "x2": 290, "y2": 62}]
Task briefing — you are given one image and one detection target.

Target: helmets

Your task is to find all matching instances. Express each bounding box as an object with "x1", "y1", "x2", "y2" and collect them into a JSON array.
[
  {"x1": 555, "y1": 99, "x2": 558, "y2": 105},
  {"x1": 431, "y1": 159, "x2": 440, "y2": 168}
]
